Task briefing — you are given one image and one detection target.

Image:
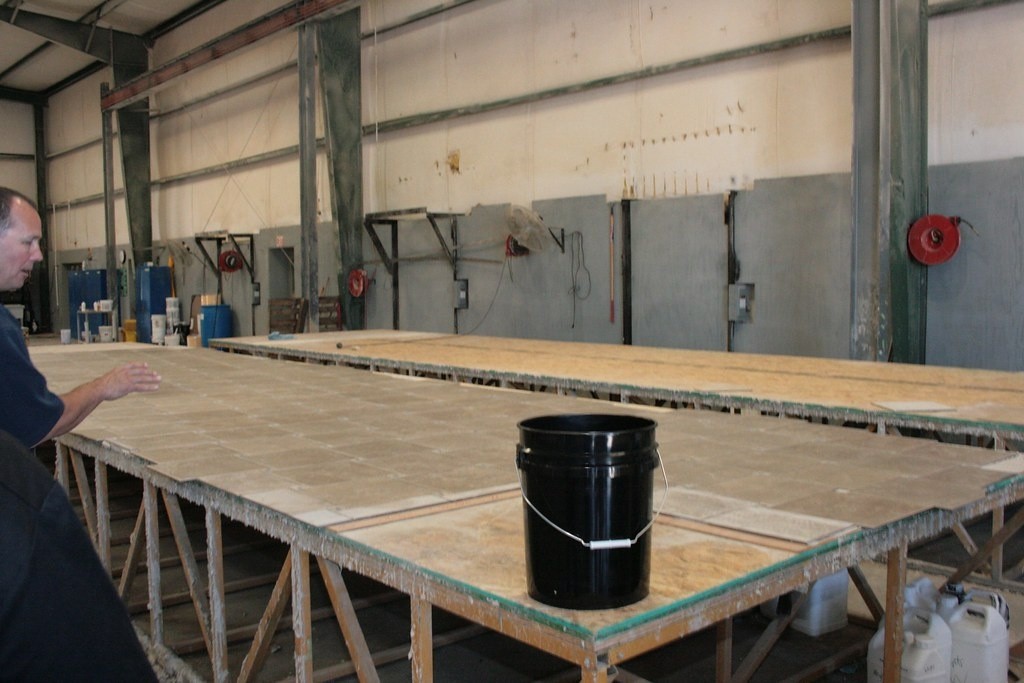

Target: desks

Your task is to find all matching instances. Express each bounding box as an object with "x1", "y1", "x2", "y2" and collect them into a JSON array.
[{"x1": 27, "y1": 328, "x2": 1024, "y2": 683}]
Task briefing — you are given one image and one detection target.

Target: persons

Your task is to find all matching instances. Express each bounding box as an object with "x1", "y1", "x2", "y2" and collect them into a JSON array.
[
  {"x1": 0, "y1": 186, "x2": 162, "y2": 451},
  {"x1": 0, "y1": 433, "x2": 160, "y2": 683}
]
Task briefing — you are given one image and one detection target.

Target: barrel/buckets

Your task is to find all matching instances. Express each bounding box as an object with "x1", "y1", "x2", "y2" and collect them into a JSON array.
[
  {"x1": 61, "y1": 329, "x2": 70, "y2": 344},
  {"x1": 867, "y1": 577, "x2": 1010, "y2": 683},
  {"x1": 99, "y1": 326, "x2": 113, "y2": 343},
  {"x1": 515, "y1": 414, "x2": 667, "y2": 611},
  {"x1": 200, "y1": 304, "x2": 233, "y2": 352},
  {"x1": 777, "y1": 567, "x2": 847, "y2": 636}
]
